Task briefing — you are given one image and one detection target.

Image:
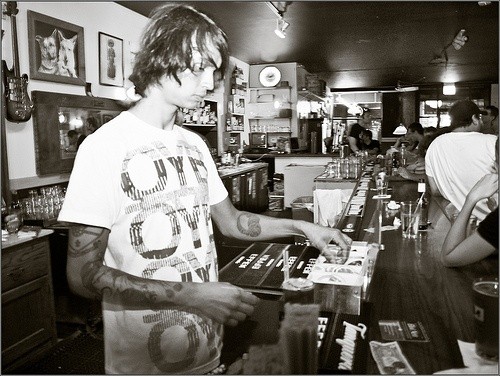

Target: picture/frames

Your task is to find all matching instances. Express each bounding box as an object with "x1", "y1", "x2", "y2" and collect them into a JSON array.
[
  {"x1": 25, "y1": 9, "x2": 85, "y2": 86},
  {"x1": 97, "y1": 31, "x2": 124, "y2": 88}
]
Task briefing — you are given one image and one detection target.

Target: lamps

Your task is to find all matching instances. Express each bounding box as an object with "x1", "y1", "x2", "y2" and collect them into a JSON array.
[
  {"x1": 267, "y1": 1, "x2": 292, "y2": 38},
  {"x1": 478, "y1": 2, "x2": 491, "y2": 6},
  {"x1": 441, "y1": 30, "x2": 468, "y2": 95}
]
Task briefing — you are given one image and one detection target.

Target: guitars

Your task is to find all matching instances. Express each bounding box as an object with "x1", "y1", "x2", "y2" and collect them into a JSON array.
[{"x1": 1, "y1": 1, "x2": 34, "y2": 124}]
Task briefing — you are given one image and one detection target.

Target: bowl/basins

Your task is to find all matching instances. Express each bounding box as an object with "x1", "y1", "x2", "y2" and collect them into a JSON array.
[{"x1": 308, "y1": 111, "x2": 317, "y2": 119}]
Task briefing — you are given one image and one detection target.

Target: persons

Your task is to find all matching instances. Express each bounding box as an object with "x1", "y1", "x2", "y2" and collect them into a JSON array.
[
  {"x1": 390, "y1": 100, "x2": 499, "y2": 226},
  {"x1": 57, "y1": 1, "x2": 353, "y2": 375},
  {"x1": 344, "y1": 124, "x2": 381, "y2": 156},
  {"x1": 440, "y1": 136, "x2": 499, "y2": 267}
]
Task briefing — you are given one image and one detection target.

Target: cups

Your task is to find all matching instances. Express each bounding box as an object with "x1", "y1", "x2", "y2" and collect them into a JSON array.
[
  {"x1": 23, "y1": 186, "x2": 67, "y2": 213},
  {"x1": 401, "y1": 201, "x2": 421, "y2": 240},
  {"x1": 375, "y1": 175, "x2": 388, "y2": 195},
  {"x1": 472, "y1": 281, "x2": 499, "y2": 361},
  {"x1": 332, "y1": 158, "x2": 361, "y2": 179},
  {"x1": 281, "y1": 278, "x2": 314, "y2": 308}
]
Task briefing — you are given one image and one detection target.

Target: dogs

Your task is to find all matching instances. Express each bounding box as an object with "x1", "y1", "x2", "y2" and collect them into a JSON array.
[{"x1": 35, "y1": 28, "x2": 78, "y2": 78}]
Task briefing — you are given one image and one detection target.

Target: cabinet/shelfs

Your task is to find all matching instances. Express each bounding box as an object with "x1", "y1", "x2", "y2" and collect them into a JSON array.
[
  {"x1": 1, "y1": 228, "x2": 58, "y2": 365},
  {"x1": 249, "y1": 86, "x2": 292, "y2": 151}
]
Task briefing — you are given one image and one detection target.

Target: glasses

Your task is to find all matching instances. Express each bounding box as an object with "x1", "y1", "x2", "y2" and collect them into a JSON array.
[{"x1": 476, "y1": 114, "x2": 483, "y2": 120}]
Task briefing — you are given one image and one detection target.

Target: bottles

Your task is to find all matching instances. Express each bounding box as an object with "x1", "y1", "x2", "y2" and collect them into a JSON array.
[
  {"x1": 415, "y1": 178, "x2": 429, "y2": 229},
  {"x1": 386, "y1": 144, "x2": 407, "y2": 168},
  {"x1": 238, "y1": 119, "x2": 242, "y2": 126},
  {"x1": 224, "y1": 150, "x2": 232, "y2": 157},
  {"x1": 226, "y1": 119, "x2": 231, "y2": 125}
]
